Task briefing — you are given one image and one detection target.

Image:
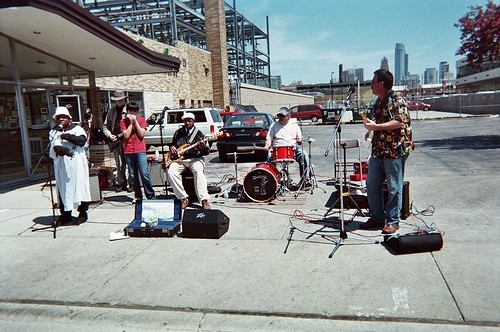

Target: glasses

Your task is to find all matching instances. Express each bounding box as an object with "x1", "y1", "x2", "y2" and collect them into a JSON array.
[{"x1": 278, "y1": 114, "x2": 286, "y2": 117}]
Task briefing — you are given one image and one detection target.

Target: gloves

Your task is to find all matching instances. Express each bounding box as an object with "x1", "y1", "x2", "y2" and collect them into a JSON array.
[
  {"x1": 61, "y1": 133, "x2": 87, "y2": 147},
  {"x1": 54, "y1": 146, "x2": 75, "y2": 158}
]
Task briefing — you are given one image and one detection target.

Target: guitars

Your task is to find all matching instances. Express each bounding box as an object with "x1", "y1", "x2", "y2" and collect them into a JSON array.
[
  {"x1": 103, "y1": 114, "x2": 155, "y2": 153},
  {"x1": 165, "y1": 130, "x2": 230, "y2": 168},
  {"x1": 359, "y1": 112, "x2": 377, "y2": 144}
]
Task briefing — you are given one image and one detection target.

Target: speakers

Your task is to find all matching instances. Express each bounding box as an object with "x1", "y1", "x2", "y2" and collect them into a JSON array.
[
  {"x1": 380, "y1": 180, "x2": 410, "y2": 218},
  {"x1": 181, "y1": 209, "x2": 230, "y2": 239}
]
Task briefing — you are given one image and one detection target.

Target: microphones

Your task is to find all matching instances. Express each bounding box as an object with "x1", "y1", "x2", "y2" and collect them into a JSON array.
[
  {"x1": 56, "y1": 124, "x2": 64, "y2": 131},
  {"x1": 346, "y1": 86, "x2": 355, "y2": 101}
]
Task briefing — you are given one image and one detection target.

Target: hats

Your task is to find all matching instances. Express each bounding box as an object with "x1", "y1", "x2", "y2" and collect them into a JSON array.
[
  {"x1": 111, "y1": 90, "x2": 128, "y2": 100},
  {"x1": 180, "y1": 113, "x2": 195, "y2": 120},
  {"x1": 277, "y1": 107, "x2": 289, "y2": 116},
  {"x1": 52, "y1": 106, "x2": 73, "y2": 119}
]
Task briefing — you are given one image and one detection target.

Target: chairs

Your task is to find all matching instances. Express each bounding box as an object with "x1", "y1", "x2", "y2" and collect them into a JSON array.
[{"x1": 334, "y1": 139, "x2": 363, "y2": 184}]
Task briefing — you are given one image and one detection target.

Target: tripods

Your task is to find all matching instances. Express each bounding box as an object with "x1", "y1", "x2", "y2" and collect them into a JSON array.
[
  {"x1": 322, "y1": 97, "x2": 380, "y2": 257},
  {"x1": 273, "y1": 142, "x2": 327, "y2": 201},
  {"x1": 31, "y1": 129, "x2": 80, "y2": 238}
]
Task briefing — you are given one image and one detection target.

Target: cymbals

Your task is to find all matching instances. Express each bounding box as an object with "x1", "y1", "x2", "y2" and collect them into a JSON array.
[
  {"x1": 237, "y1": 145, "x2": 269, "y2": 151},
  {"x1": 302, "y1": 136, "x2": 316, "y2": 142}
]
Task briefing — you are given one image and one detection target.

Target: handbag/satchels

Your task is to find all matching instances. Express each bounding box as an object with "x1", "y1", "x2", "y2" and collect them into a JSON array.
[{"x1": 384, "y1": 231, "x2": 443, "y2": 255}]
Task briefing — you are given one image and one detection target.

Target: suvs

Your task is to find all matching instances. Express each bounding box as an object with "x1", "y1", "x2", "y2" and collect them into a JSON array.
[{"x1": 141, "y1": 107, "x2": 224, "y2": 151}]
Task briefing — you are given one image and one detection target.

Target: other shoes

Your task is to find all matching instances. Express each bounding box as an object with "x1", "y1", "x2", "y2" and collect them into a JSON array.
[
  {"x1": 202, "y1": 200, "x2": 210, "y2": 209},
  {"x1": 359, "y1": 219, "x2": 385, "y2": 229},
  {"x1": 127, "y1": 187, "x2": 134, "y2": 193},
  {"x1": 382, "y1": 224, "x2": 399, "y2": 233},
  {"x1": 181, "y1": 198, "x2": 189, "y2": 208},
  {"x1": 131, "y1": 198, "x2": 142, "y2": 204},
  {"x1": 116, "y1": 186, "x2": 127, "y2": 191},
  {"x1": 56, "y1": 213, "x2": 71, "y2": 225},
  {"x1": 75, "y1": 211, "x2": 88, "y2": 224}
]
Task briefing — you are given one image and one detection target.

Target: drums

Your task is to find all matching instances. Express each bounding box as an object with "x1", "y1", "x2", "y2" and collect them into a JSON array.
[
  {"x1": 242, "y1": 162, "x2": 282, "y2": 203},
  {"x1": 273, "y1": 146, "x2": 294, "y2": 166}
]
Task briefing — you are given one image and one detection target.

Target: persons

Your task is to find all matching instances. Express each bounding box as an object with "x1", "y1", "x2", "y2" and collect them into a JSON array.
[
  {"x1": 360, "y1": 68, "x2": 415, "y2": 234},
  {"x1": 263, "y1": 106, "x2": 312, "y2": 189},
  {"x1": 169, "y1": 112, "x2": 211, "y2": 211},
  {"x1": 120, "y1": 102, "x2": 156, "y2": 203},
  {"x1": 147, "y1": 115, "x2": 157, "y2": 125},
  {"x1": 101, "y1": 90, "x2": 134, "y2": 193},
  {"x1": 46, "y1": 105, "x2": 90, "y2": 225},
  {"x1": 78, "y1": 108, "x2": 95, "y2": 155}
]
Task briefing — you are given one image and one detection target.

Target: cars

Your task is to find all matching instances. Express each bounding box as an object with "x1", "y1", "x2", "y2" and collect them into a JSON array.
[
  {"x1": 218, "y1": 112, "x2": 277, "y2": 162},
  {"x1": 408, "y1": 101, "x2": 432, "y2": 111},
  {"x1": 276, "y1": 104, "x2": 325, "y2": 123},
  {"x1": 219, "y1": 111, "x2": 266, "y2": 127}
]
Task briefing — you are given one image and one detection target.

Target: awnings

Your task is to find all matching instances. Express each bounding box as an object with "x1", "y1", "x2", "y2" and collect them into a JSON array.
[{"x1": 4, "y1": 0, "x2": 181, "y2": 81}]
